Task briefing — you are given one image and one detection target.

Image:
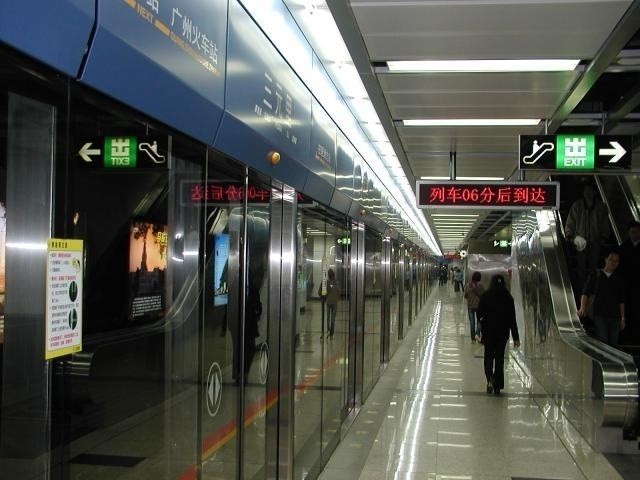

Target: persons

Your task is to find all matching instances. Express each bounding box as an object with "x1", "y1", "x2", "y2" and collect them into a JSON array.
[
  {"x1": 326, "y1": 270, "x2": 341, "y2": 340},
  {"x1": 436, "y1": 264, "x2": 466, "y2": 291},
  {"x1": 479, "y1": 275, "x2": 521, "y2": 396},
  {"x1": 463, "y1": 272, "x2": 485, "y2": 344},
  {"x1": 577, "y1": 248, "x2": 627, "y2": 350},
  {"x1": 619, "y1": 219, "x2": 639, "y2": 341},
  {"x1": 220, "y1": 272, "x2": 261, "y2": 387},
  {"x1": 564, "y1": 182, "x2": 612, "y2": 272}
]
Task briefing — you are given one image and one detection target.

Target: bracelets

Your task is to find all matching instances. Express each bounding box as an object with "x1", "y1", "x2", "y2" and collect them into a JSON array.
[{"x1": 620, "y1": 316, "x2": 626, "y2": 320}]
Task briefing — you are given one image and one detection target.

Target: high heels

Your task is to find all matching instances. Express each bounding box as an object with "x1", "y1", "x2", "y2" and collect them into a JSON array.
[
  {"x1": 487, "y1": 374, "x2": 501, "y2": 395},
  {"x1": 471, "y1": 334, "x2": 481, "y2": 343}
]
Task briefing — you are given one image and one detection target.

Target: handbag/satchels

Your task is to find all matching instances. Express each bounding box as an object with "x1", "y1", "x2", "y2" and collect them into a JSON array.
[
  {"x1": 476, "y1": 304, "x2": 489, "y2": 326},
  {"x1": 575, "y1": 280, "x2": 600, "y2": 320},
  {"x1": 572, "y1": 236, "x2": 587, "y2": 252}
]
